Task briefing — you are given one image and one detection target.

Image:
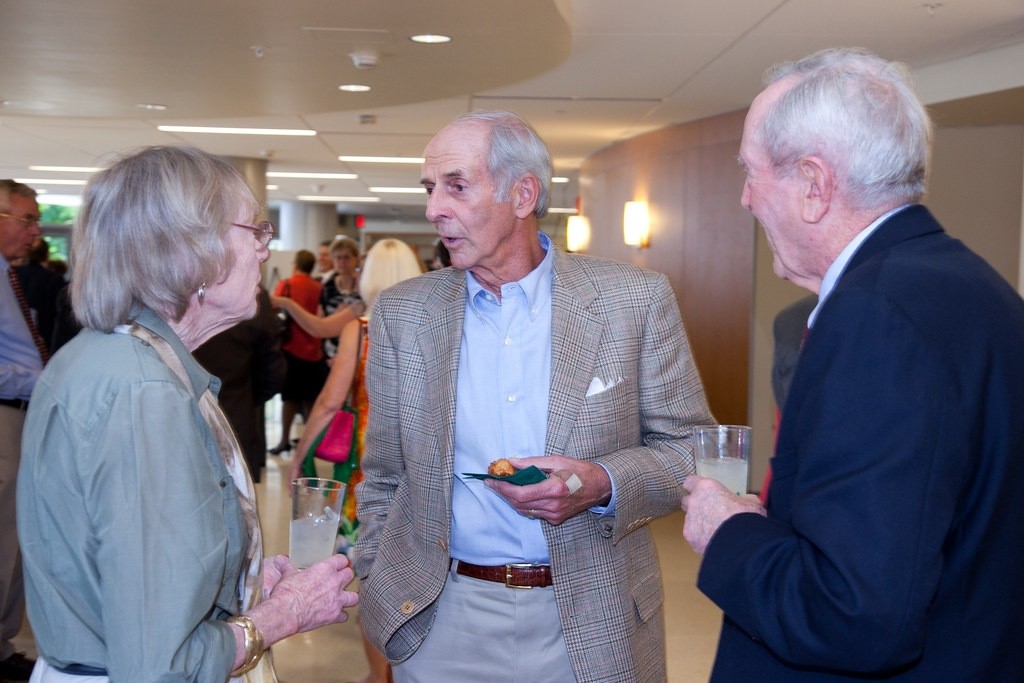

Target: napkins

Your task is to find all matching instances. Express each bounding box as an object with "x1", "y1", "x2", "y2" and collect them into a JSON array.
[{"x1": 462, "y1": 466, "x2": 548, "y2": 487}]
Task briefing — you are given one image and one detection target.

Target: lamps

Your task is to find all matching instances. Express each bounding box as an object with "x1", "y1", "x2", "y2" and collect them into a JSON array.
[
  {"x1": 564, "y1": 214, "x2": 588, "y2": 249},
  {"x1": 623, "y1": 201, "x2": 647, "y2": 248}
]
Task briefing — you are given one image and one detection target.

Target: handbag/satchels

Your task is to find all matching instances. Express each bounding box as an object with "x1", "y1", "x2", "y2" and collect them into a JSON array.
[
  {"x1": 283, "y1": 315, "x2": 294, "y2": 343},
  {"x1": 314, "y1": 318, "x2": 363, "y2": 462}
]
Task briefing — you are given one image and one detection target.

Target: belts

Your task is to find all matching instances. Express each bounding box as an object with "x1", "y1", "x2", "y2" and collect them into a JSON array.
[
  {"x1": 449, "y1": 558, "x2": 553, "y2": 589},
  {"x1": 229, "y1": 220, "x2": 274, "y2": 249},
  {"x1": 0, "y1": 398, "x2": 29, "y2": 412}
]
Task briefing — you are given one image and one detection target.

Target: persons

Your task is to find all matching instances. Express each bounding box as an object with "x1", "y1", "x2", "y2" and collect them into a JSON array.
[
  {"x1": 683, "y1": 45, "x2": 1024, "y2": 683},
  {"x1": 351, "y1": 110, "x2": 720, "y2": 683},
  {"x1": 192, "y1": 235, "x2": 455, "y2": 563},
  {"x1": 15, "y1": 143, "x2": 359, "y2": 683},
  {"x1": 0, "y1": 179, "x2": 50, "y2": 683},
  {"x1": 13, "y1": 239, "x2": 84, "y2": 355}
]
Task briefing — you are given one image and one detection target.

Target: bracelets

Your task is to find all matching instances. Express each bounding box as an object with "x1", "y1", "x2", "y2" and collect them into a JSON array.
[{"x1": 227, "y1": 616, "x2": 264, "y2": 678}]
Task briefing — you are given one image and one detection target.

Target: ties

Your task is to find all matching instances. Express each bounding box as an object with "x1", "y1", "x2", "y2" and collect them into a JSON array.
[{"x1": 8, "y1": 265, "x2": 49, "y2": 366}]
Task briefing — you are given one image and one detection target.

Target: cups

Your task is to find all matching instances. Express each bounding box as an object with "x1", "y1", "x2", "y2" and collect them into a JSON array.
[
  {"x1": 289, "y1": 477, "x2": 347, "y2": 571},
  {"x1": 692, "y1": 424, "x2": 753, "y2": 497}
]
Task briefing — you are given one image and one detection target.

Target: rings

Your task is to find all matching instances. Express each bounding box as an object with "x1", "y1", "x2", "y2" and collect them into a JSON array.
[{"x1": 528, "y1": 510, "x2": 535, "y2": 520}]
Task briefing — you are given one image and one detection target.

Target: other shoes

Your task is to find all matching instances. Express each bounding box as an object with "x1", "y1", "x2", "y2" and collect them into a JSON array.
[{"x1": 270, "y1": 443, "x2": 291, "y2": 456}]
